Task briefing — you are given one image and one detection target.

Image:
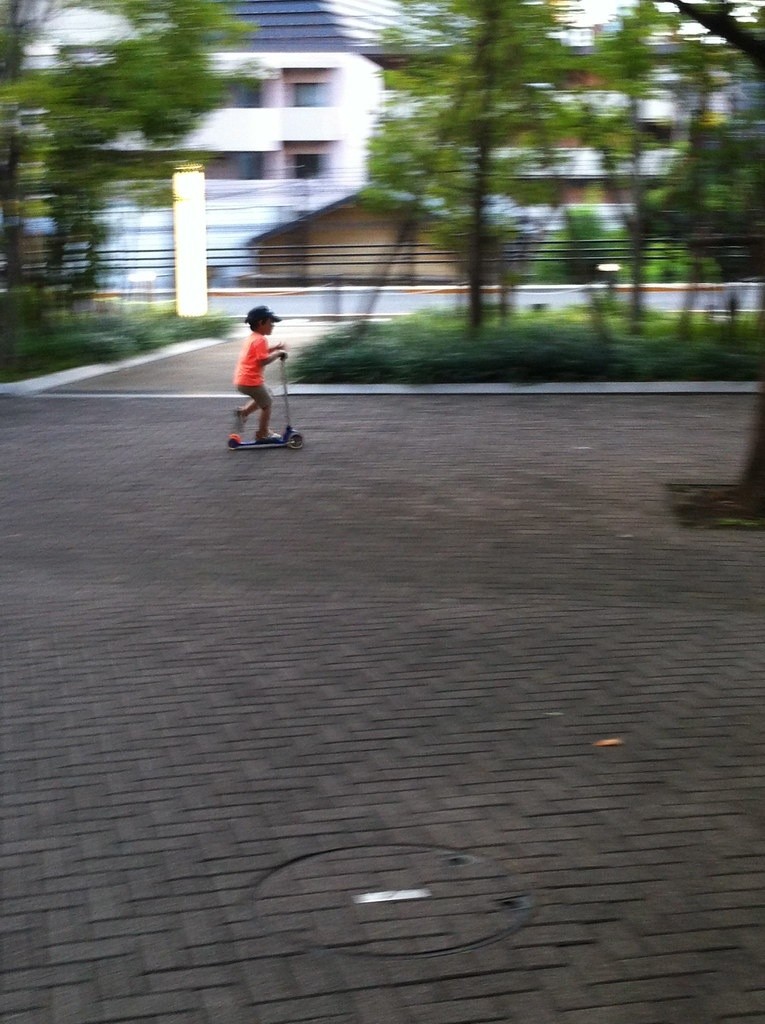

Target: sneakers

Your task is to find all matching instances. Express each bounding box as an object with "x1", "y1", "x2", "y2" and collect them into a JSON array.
[
  {"x1": 256, "y1": 429, "x2": 282, "y2": 441},
  {"x1": 234, "y1": 408, "x2": 247, "y2": 432}
]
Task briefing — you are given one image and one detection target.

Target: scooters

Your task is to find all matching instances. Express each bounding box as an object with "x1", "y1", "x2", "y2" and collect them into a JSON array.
[{"x1": 228, "y1": 347, "x2": 303, "y2": 451}]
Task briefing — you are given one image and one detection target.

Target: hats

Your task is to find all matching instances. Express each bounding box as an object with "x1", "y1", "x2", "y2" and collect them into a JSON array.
[{"x1": 245, "y1": 306, "x2": 281, "y2": 323}]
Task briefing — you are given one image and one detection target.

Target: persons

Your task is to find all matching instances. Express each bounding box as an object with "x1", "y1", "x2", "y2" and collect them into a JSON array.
[{"x1": 235, "y1": 306, "x2": 287, "y2": 442}]
